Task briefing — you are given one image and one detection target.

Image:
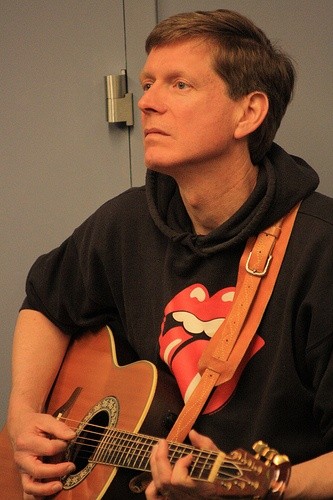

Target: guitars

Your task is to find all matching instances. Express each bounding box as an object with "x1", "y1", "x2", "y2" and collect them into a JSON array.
[{"x1": 0, "y1": 325, "x2": 292, "y2": 500}]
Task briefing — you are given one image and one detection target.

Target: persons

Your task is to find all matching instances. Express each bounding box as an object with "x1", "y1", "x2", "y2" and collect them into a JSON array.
[{"x1": 6, "y1": 9, "x2": 333, "y2": 500}]
